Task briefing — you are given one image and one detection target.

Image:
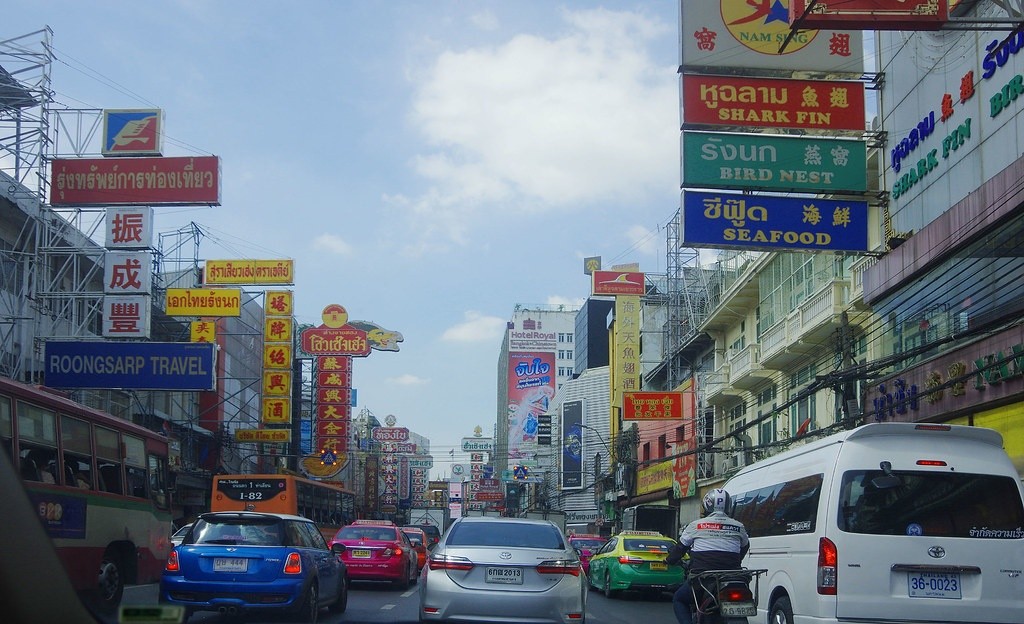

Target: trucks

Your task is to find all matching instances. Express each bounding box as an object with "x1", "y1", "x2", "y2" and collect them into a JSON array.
[
  {"x1": 622, "y1": 504, "x2": 678, "y2": 542},
  {"x1": 410, "y1": 507, "x2": 451, "y2": 536},
  {"x1": 526, "y1": 510, "x2": 567, "y2": 534}
]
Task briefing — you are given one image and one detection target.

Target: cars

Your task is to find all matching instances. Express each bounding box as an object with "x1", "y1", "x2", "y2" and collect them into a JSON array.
[
  {"x1": 160, "y1": 510, "x2": 349, "y2": 624},
  {"x1": 587, "y1": 530, "x2": 687, "y2": 598},
  {"x1": 398, "y1": 527, "x2": 428, "y2": 570},
  {"x1": 327, "y1": 520, "x2": 418, "y2": 589},
  {"x1": 419, "y1": 516, "x2": 588, "y2": 624},
  {"x1": 568, "y1": 534, "x2": 608, "y2": 573}
]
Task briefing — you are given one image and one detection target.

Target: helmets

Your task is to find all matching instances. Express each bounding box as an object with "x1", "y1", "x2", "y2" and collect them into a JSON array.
[{"x1": 702, "y1": 488, "x2": 732, "y2": 515}]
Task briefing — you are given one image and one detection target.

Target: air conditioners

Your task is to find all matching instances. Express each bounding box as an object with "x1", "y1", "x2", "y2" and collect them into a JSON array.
[
  {"x1": 722, "y1": 458, "x2": 734, "y2": 474},
  {"x1": 722, "y1": 348, "x2": 738, "y2": 363}
]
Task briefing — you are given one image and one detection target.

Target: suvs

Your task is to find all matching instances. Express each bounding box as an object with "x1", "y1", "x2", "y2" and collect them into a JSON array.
[{"x1": 403, "y1": 524, "x2": 440, "y2": 551}]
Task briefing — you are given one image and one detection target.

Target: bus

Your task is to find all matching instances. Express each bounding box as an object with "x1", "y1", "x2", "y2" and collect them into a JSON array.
[
  {"x1": 0, "y1": 377, "x2": 172, "y2": 614},
  {"x1": 210, "y1": 473, "x2": 355, "y2": 541}
]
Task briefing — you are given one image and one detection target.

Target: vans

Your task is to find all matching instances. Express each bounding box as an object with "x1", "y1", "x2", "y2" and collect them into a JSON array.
[{"x1": 721, "y1": 422, "x2": 1024, "y2": 624}]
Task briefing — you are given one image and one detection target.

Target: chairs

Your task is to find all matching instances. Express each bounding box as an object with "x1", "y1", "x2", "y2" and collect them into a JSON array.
[
  {"x1": 219, "y1": 524, "x2": 240, "y2": 540},
  {"x1": 133, "y1": 478, "x2": 145, "y2": 497},
  {"x1": 101, "y1": 466, "x2": 122, "y2": 493},
  {"x1": 19, "y1": 458, "x2": 41, "y2": 481},
  {"x1": 76, "y1": 469, "x2": 106, "y2": 493},
  {"x1": 48, "y1": 465, "x2": 75, "y2": 488}
]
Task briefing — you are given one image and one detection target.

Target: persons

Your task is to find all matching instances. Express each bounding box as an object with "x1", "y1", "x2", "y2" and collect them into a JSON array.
[
  {"x1": 666, "y1": 488, "x2": 751, "y2": 624},
  {"x1": 27, "y1": 450, "x2": 55, "y2": 483},
  {"x1": 65, "y1": 459, "x2": 92, "y2": 489}
]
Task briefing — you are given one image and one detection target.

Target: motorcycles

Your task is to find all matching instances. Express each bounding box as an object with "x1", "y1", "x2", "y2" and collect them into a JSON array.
[{"x1": 664, "y1": 547, "x2": 768, "y2": 624}]
